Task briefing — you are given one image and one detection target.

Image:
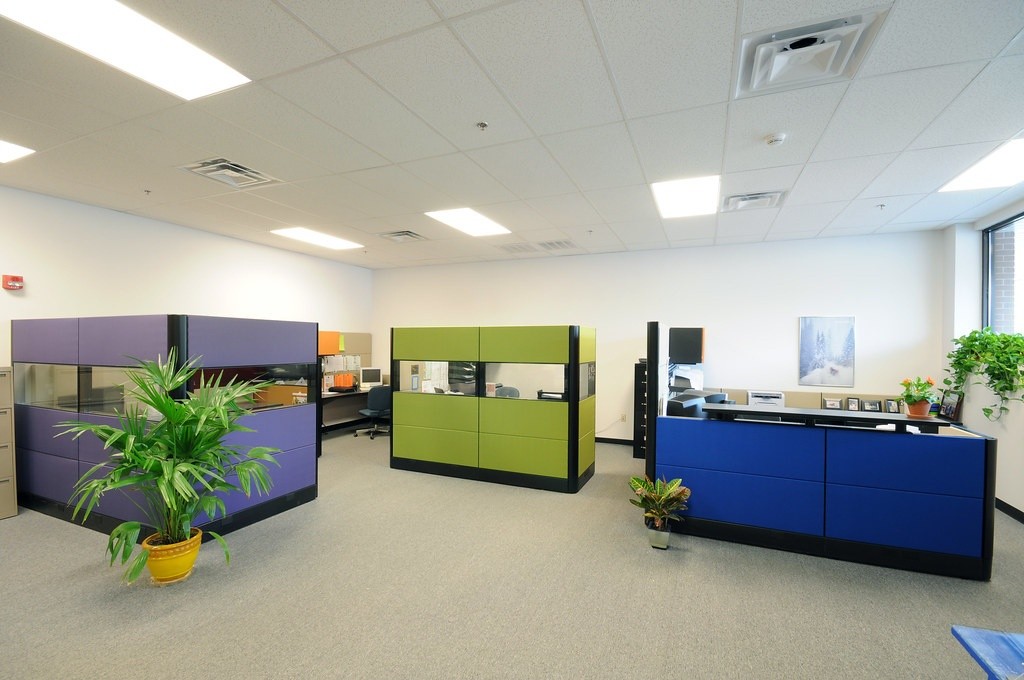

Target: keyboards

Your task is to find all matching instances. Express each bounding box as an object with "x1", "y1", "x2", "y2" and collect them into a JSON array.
[{"x1": 360, "y1": 388, "x2": 372, "y2": 391}]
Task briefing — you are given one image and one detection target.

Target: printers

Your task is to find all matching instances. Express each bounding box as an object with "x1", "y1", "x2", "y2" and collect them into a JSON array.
[{"x1": 747, "y1": 391, "x2": 785, "y2": 421}]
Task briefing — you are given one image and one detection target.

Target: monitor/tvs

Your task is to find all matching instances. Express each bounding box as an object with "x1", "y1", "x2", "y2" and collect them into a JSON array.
[{"x1": 359, "y1": 367, "x2": 383, "y2": 388}]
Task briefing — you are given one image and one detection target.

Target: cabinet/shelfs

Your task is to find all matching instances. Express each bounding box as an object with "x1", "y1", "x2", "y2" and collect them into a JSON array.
[
  {"x1": 0, "y1": 366, "x2": 18, "y2": 520},
  {"x1": 633, "y1": 363, "x2": 647, "y2": 459}
]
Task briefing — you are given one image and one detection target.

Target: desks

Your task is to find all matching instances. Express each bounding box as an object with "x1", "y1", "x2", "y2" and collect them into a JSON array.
[{"x1": 293, "y1": 383, "x2": 388, "y2": 435}]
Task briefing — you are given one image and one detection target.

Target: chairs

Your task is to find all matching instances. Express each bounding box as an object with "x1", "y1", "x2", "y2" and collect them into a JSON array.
[{"x1": 354, "y1": 386, "x2": 391, "y2": 439}]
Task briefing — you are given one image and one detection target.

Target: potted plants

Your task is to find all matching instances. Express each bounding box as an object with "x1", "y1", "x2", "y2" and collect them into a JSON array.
[
  {"x1": 53, "y1": 345, "x2": 288, "y2": 587},
  {"x1": 627, "y1": 474, "x2": 691, "y2": 550},
  {"x1": 938, "y1": 326, "x2": 1024, "y2": 423},
  {"x1": 894, "y1": 375, "x2": 936, "y2": 419}
]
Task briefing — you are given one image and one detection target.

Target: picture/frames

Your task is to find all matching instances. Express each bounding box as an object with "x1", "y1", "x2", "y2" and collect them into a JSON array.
[
  {"x1": 799, "y1": 316, "x2": 855, "y2": 388},
  {"x1": 823, "y1": 396, "x2": 904, "y2": 413},
  {"x1": 937, "y1": 390, "x2": 965, "y2": 421}
]
